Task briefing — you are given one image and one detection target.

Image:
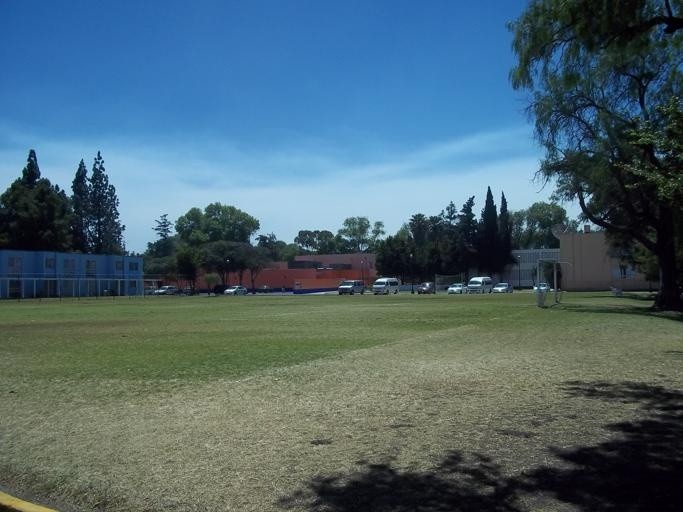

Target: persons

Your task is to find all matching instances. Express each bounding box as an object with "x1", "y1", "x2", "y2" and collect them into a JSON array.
[{"x1": 619, "y1": 255, "x2": 627, "y2": 278}]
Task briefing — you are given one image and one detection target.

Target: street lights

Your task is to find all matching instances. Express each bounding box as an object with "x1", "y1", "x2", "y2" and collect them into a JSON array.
[{"x1": 409, "y1": 252, "x2": 414, "y2": 293}]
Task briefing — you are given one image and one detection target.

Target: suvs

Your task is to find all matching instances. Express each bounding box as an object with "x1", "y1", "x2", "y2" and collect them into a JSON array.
[{"x1": 338, "y1": 280, "x2": 364, "y2": 295}]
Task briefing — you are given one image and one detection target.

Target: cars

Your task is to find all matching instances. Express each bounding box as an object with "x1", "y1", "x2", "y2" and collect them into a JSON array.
[
  {"x1": 224, "y1": 286, "x2": 247, "y2": 296},
  {"x1": 144, "y1": 285, "x2": 197, "y2": 295},
  {"x1": 448, "y1": 277, "x2": 513, "y2": 294},
  {"x1": 417, "y1": 282, "x2": 435, "y2": 294},
  {"x1": 533, "y1": 283, "x2": 550, "y2": 291}
]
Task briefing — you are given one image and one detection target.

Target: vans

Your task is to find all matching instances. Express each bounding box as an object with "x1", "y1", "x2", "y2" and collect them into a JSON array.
[{"x1": 371, "y1": 278, "x2": 399, "y2": 295}]
